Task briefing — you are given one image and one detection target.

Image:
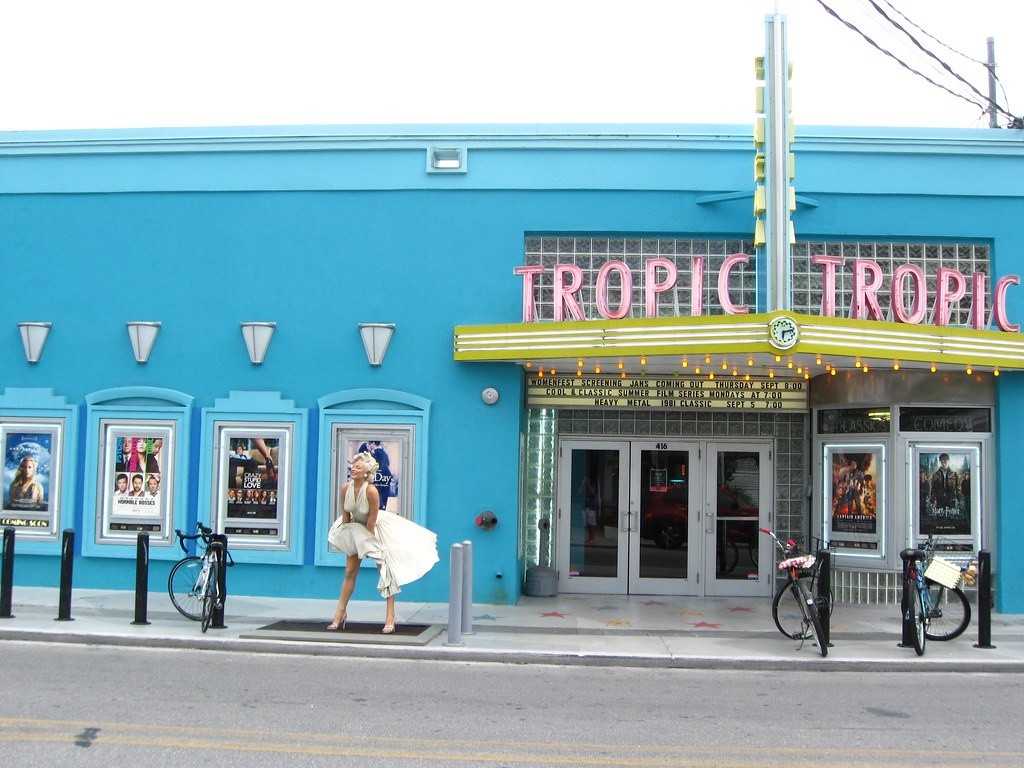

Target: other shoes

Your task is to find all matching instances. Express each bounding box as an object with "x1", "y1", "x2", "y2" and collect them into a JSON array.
[{"x1": 266, "y1": 457, "x2": 275, "y2": 481}]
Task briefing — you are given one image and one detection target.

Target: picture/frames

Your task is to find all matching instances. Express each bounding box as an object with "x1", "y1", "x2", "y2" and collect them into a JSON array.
[
  {"x1": 218, "y1": 426, "x2": 287, "y2": 545},
  {"x1": 0, "y1": 421, "x2": 65, "y2": 542},
  {"x1": 908, "y1": 443, "x2": 982, "y2": 559},
  {"x1": 94, "y1": 418, "x2": 177, "y2": 546},
  {"x1": 823, "y1": 443, "x2": 888, "y2": 561},
  {"x1": 333, "y1": 422, "x2": 412, "y2": 551}
]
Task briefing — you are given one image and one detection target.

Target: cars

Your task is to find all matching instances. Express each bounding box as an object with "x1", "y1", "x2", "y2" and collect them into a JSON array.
[{"x1": 640, "y1": 480, "x2": 759, "y2": 551}]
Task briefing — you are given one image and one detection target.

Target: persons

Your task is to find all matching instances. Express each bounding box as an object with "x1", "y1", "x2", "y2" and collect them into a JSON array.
[
  {"x1": 929, "y1": 454, "x2": 960, "y2": 516},
  {"x1": 136, "y1": 438, "x2": 147, "y2": 473},
  {"x1": 115, "y1": 474, "x2": 128, "y2": 496},
  {"x1": 960, "y1": 472, "x2": 970, "y2": 514},
  {"x1": 581, "y1": 475, "x2": 598, "y2": 545},
  {"x1": 145, "y1": 475, "x2": 159, "y2": 498},
  {"x1": 146, "y1": 438, "x2": 162, "y2": 472},
  {"x1": 9, "y1": 457, "x2": 44, "y2": 504},
  {"x1": 229, "y1": 446, "x2": 258, "y2": 489},
  {"x1": 252, "y1": 490, "x2": 259, "y2": 501},
  {"x1": 260, "y1": 490, "x2": 268, "y2": 502},
  {"x1": 838, "y1": 461, "x2": 865, "y2": 517},
  {"x1": 129, "y1": 474, "x2": 144, "y2": 497},
  {"x1": 833, "y1": 486, "x2": 846, "y2": 514},
  {"x1": 268, "y1": 490, "x2": 277, "y2": 503},
  {"x1": 228, "y1": 489, "x2": 236, "y2": 502},
  {"x1": 235, "y1": 490, "x2": 243, "y2": 501},
  {"x1": 368, "y1": 441, "x2": 392, "y2": 511},
  {"x1": 243, "y1": 489, "x2": 252, "y2": 501},
  {"x1": 116, "y1": 437, "x2": 130, "y2": 471},
  {"x1": 358, "y1": 443, "x2": 371, "y2": 453},
  {"x1": 864, "y1": 474, "x2": 872, "y2": 489},
  {"x1": 325, "y1": 451, "x2": 397, "y2": 635},
  {"x1": 250, "y1": 438, "x2": 276, "y2": 481},
  {"x1": 921, "y1": 471, "x2": 930, "y2": 516}
]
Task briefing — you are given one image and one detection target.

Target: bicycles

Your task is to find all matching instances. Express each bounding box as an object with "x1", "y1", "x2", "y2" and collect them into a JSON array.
[
  {"x1": 899, "y1": 524, "x2": 975, "y2": 657},
  {"x1": 716, "y1": 525, "x2": 761, "y2": 575},
  {"x1": 759, "y1": 528, "x2": 835, "y2": 658},
  {"x1": 167, "y1": 521, "x2": 225, "y2": 634}
]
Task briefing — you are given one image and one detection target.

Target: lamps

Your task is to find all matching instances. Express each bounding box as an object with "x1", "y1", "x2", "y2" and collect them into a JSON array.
[{"x1": 523, "y1": 351, "x2": 1003, "y2": 385}]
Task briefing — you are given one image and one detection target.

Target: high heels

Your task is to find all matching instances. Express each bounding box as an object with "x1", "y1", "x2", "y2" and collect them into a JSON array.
[
  {"x1": 327, "y1": 611, "x2": 348, "y2": 629},
  {"x1": 383, "y1": 615, "x2": 396, "y2": 633}
]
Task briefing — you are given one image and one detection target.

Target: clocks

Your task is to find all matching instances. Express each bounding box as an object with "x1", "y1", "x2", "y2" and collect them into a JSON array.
[{"x1": 769, "y1": 315, "x2": 801, "y2": 352}]
